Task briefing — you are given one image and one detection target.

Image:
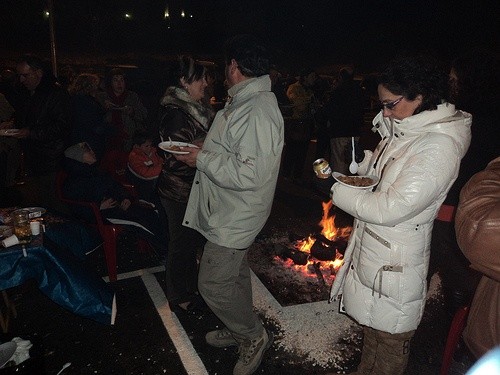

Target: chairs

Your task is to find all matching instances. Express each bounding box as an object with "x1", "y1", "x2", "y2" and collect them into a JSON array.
[
  {"x1": 55, "y1": 147, "x2": 153, "y2": 284},
  {"x1": 424, "y1": 204, "x2": 470, "y2": 375}
]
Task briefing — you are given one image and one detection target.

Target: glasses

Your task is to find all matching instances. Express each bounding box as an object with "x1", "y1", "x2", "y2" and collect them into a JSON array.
[{"x1": 377, "y1": 96, "x2": 404, "y2": 110}]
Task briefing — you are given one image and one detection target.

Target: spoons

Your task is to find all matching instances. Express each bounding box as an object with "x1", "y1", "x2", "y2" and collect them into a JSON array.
[{"x1": 348, "y1": 136, "x2": 359, "y2": 174}]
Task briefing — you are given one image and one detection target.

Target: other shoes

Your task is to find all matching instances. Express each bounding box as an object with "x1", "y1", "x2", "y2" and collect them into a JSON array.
[
  {"x1": 280, "y1": 175, "x2": 303, "y2": 186},
  {"x1": 171, "y1": 297, "x2": 211, "y2": 320},
  {"x1": 290, "y1": 201, "x2": 312, "y2": 217}
]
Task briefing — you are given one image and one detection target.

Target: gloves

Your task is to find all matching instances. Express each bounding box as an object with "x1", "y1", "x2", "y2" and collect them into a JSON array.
[
  {"x1": 320, "y1": 176, "x2": 337, "y2": 195},
  {"x1": 344, "y1": 142, "x2": 365, "y2": 164}
]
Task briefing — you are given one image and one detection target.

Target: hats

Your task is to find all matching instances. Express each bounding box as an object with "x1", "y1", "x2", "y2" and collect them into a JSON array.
[{"x1": 65, "y1": 141, "x2": 96, "y2": 163}]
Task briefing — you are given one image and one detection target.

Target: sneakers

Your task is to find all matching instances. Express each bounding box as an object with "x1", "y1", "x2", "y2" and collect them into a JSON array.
[{"x1": 233, "y1": 326, "x2": 274, "y2": 375}]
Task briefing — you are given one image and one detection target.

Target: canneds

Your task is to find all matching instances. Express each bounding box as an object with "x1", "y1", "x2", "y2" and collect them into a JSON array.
[{"x1": 312, "y1": 158, "x2": 332, "y2": 179}]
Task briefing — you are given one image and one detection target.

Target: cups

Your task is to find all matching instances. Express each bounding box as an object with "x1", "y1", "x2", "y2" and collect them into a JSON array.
[
  {"x1": 2, "y1": 234, "x2": 19, "y2": 248},
  {"x1": 12, "y1": 209, "x2": 33, "y2": 242},
  {"x1": 29, "y1": 221, "x2": 42, "y2": 238}
]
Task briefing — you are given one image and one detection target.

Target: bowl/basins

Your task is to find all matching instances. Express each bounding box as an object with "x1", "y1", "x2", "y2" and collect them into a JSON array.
[{"x1": 332, "y1": 171, "x2": 380, "y2": 190}]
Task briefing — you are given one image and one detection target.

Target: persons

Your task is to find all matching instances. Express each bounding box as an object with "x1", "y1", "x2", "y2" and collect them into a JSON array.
[
  {"x1": 157, "y1": 55, "x2": 216, "y2": 317},
  {"x1": 175, "y1": 33, "x2": 285, "y2": 375},
  {"x1": 329, "y1": 65, "x2": 473, "y2": 375},
  {"x1": 0, "y1": 51, "x2": 383, "y2": 265},
  {"x1": 447, "y1": 154, "x2": 500, "y2": 375}
]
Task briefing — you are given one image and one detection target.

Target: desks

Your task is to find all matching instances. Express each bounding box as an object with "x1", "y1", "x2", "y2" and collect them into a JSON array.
[{"x1": 0, "y1": 215, "x2": 121, "y2": 333}]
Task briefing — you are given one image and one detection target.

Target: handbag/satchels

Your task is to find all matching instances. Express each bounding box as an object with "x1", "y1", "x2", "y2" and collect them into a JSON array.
[
  {"x1": 206, "y1": 318, "x2": 264, "y2": 348},
  {"x1": 286, "y1": 118, "x2": 314, "y2": 143}
]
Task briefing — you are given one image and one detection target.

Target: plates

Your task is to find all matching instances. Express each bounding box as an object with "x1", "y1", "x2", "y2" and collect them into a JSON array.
[
  {"x1": 23, "y1": 207, "x2": 48, "y2": 218},
  {"x1": 0, "y1": 226, "x2": 11, "y2": 238},
  {"x1": 158, "y1": 141, "x2": 199, "y2": 155}
]
